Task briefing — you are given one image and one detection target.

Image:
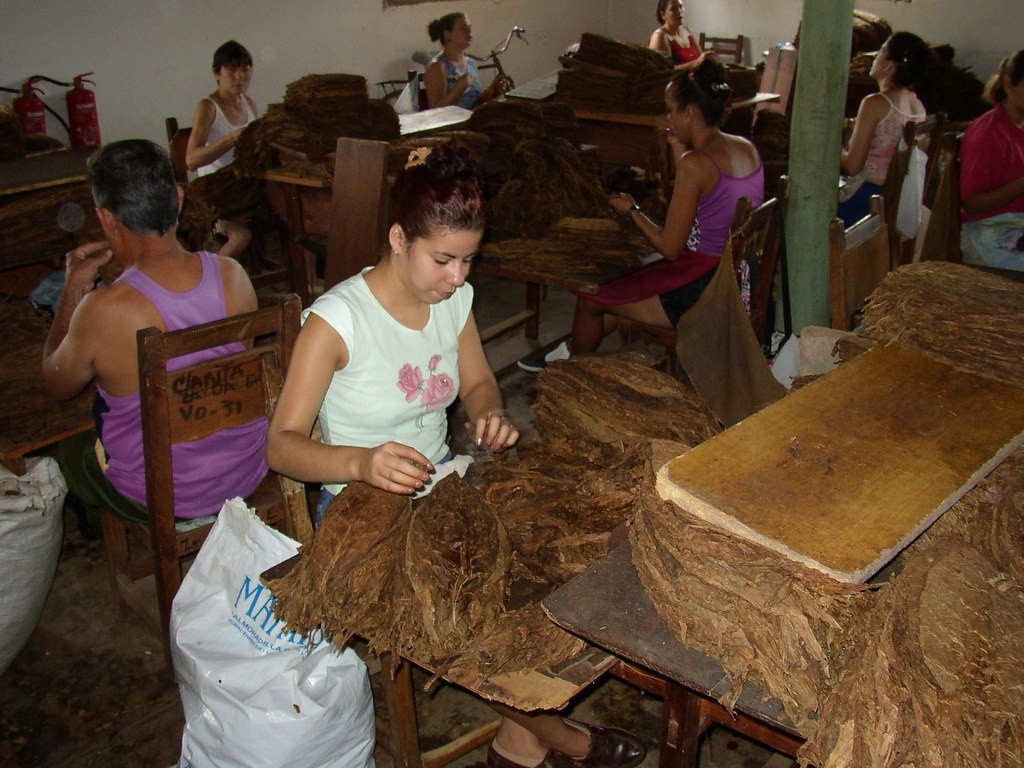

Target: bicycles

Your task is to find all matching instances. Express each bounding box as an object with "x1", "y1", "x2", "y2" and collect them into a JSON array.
[{"x1": 375, "y1": 25, "x2": 529, "y2": 111}]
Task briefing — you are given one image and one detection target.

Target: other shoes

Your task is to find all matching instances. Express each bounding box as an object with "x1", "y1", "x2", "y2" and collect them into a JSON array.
[{"x1": 517, "y1": 354, "x2": 550, "y2": 372}]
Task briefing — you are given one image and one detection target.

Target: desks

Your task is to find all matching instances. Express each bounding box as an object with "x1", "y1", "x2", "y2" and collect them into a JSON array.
[{"x1": 0, "y1": 73, "x2": 1024, "y2": 768}]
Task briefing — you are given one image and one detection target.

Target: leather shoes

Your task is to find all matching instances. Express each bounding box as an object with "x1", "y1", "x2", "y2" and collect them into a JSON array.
[
  {"x1": 537, "y1": 713, "x2": 647, "y2": 768},
  {"x1": 487, "y1": 734, "x2": 588, "y2": 768}
]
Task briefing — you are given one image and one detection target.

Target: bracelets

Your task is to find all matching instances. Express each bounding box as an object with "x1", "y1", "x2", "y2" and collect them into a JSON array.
[
  {"x1": 843, "y1": 118, "x2": 854, "y2": 134},
  {"x1": 626, "y1": 205, "x2": 641, "y2": 218}
]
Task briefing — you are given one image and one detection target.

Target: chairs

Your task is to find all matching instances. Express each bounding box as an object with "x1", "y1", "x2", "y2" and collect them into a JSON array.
[
  {"x1": 98, "y1": 292, "x2": 317, "y2": 683},
  {"x1": 259, "y1": 359, "x2": 501, "y2": 768},
  {"x1": 699, "y1": 32, "x2": 744, "y2": 64},
  {"x1": 880, "y1": 111, "x2": 948, "y2": 271},
  {"x1": 165, "y1": 117, "x2": 193, "y2": 169},
  {"x1": 829, "y1": 193, "x2": 891, "y2": 333},
  {"x1": 941, "y1": 131, "x2": 968, "y2": 264},
  {"x1": 408, "y1": 70, "x2": 429, "y2": 111},
  {"x1": 616, "y1": 173, "x2": 790, "y2": 382}
]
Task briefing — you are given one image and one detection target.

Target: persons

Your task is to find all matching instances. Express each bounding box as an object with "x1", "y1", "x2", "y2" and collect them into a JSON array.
[
  {"x1": 424, "y1": 12, "x2": 511, "y2": 109},
  {"x1": 265, "y1": 138, "x2": 648, "y2": 768},
  {"x1": 185, "y1": 40, "x2": 259, "y2": 257},
  {"x1": 956, "y1": 49, "x2": 1024, "y2": 272},
  {"x1": 517, "y1": 59, "x2": 765, "y2": 373},
  {"x1": 649, "y1": 0, "x2": 715, "y2": 72},
  {"x1": 837, "y1": 31, "x2": 927, "y2": 229},
  {"x1": 42, "y1": 139, "x2": 270, "y2": 542}
]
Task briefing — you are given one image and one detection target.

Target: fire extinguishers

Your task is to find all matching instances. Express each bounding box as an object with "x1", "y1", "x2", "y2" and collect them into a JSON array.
[
  {"x1": 12, "y1": 75, "x2": 47, "y2": 139},
  {"x1": 65, "y1": 71, "x2": 103, "y2": 173}
]
